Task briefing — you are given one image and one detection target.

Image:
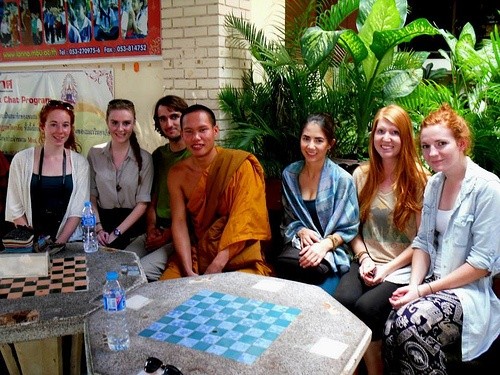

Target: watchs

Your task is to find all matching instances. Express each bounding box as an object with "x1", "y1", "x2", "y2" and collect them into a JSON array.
[{"x1": 114, "y1": 229, "x2": 121, "y2": 236}]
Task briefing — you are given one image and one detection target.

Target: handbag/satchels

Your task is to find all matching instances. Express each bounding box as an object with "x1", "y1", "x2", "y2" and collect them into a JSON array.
[
  {"x1": 277, "y1": 246, "x2": 331, "y2": 286},
  {"x1": 2, "y1": 228, "x2": 35, "y2": 252}
]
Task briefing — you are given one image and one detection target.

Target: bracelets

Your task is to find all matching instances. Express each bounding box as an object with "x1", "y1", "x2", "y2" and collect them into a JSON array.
[
  {"x1": 359, "y1": 252, "x2": 370, "y2": 265},
  {"x1": 427, "y1": 283, "x2": 434, "y2": 294},
  {"x1": 417, "y1": 285, "x2": 422, "y2": 297}
]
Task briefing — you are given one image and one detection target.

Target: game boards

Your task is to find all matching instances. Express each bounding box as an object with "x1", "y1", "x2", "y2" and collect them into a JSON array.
[
  {"x1": 0, "y1": 256, "x2": 87, "y2": 299},
  {"x1": 137, "y1": 289, "x2": 302, "y2": 367}
]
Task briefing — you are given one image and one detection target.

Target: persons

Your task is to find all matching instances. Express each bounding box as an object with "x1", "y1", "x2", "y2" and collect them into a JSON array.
[
  {"x1": 5, "y1": 100, "x2": 90, "y2": 245},
  {"x1": 87, "y1": 99, "x2": 153, "y2": 251},
  {"x1": 160, "y1": 104, "x2": 276, "y2": 282},
  {"x1": 333, "y1": 106, "x2": 431, "y2": 375},
  {"x1": 121, "y1": 94, "x2": 191, "y2": 282},
  {"x1": 385, "y1": 103, "x2": 500, "y2": 375},
  {"x1": 280, "y1": 113, "x2": 360, "y2": 298},
  {"x1": 0, "y1": 0, "x2": 149, "y2": 48}
]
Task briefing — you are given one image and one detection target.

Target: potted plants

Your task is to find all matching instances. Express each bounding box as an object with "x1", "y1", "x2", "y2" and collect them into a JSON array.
[
  {"x1": 221, "y1": 65, "x2": 303, "y2": 212},
  {"x1": 299, "y1": 0, "x2": 443, "y2": 175}
]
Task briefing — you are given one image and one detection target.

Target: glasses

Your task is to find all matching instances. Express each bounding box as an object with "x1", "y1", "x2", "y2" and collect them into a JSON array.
[
  {"x1": 109, "y1": 99, "x2": 131, "y2": 105},
  {"x1": 41, "y1": 100, "x2": 74, "y2": 116},
  {"x1": 143, "y1": 356, "x2": 184, "y2": 375}
]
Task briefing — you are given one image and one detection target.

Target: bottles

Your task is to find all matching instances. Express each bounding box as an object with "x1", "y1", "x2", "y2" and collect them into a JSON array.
[
  {"x1": 103, "y1": 271, "x2": 130, "y2": 351},
  {"x1": 81, "y1": 200, "x2": 98, "y2": 253}
]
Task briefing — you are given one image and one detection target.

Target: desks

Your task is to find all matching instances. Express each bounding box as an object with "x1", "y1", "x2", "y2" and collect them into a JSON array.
[{"x1": 0, "y1": 243, "x2": 372, "y2": 375}]
